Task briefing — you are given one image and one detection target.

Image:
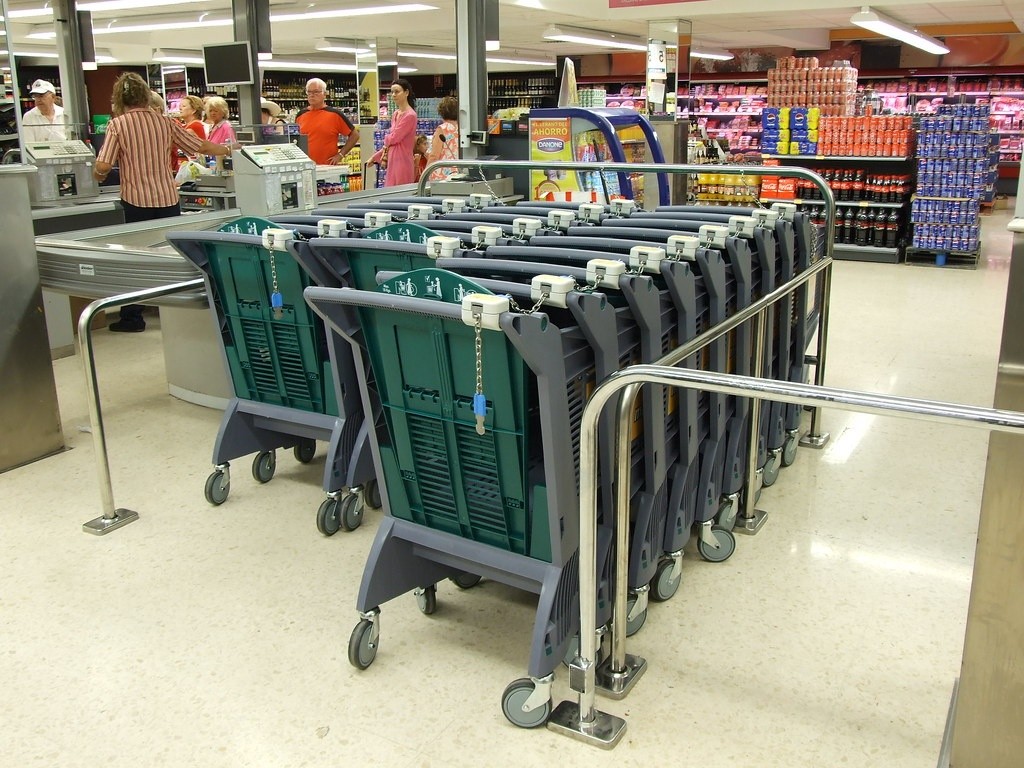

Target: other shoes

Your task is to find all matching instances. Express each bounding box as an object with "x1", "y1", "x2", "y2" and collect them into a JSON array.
[{"x1": 109, "y1": 319, "x2": 146, "y2": 332}]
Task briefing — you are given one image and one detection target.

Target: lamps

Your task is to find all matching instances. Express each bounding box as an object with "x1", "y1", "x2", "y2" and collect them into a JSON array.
[
  {"x1": 542, "y1": 23, "x2": 735, "y2": 62},
  {"x1": 149, "y1": 47, "x2": 418, "y2": 74},
  {"x1": 313, "y1": 36, "x2": 558, "y2": 65},
  {"x1": 849, "y1": 7, "x2": 952, "y2": 56},
  {"x1": 0, "y1": 42, "x2": 115, "y2": 60}
]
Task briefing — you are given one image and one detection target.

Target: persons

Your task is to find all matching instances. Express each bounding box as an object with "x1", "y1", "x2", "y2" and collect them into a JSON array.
[
  {"x1": 260, "y1": 97, "x2": 284, "y2": 134},
  {"x1": 174, "y1": 96, "x2": 206, "y2": 173},
  {"x1": 365, "y1": 79, "x2": 418, "y2": 187},
  {"x1": 22, "y1": 79, "x2": 66, "y2": 143},
  {"x1": 413, "y1": 133, "x2": 430, "y2": 182},
  {"x1": 94, "y1": 71, "x2": 242, "y2": 332},
  {"x1": 294, "y1": 77, "x2": 360, "y2": 165},
  {"x1": 149, "y1": 89, "x2": 183, "y2": 171},
  {"x1": 206, "y1": 96, "x2": 236, "y2": 144},
  {"x1": 426, "y1": 97, "x2": 459, "y2": 181}
]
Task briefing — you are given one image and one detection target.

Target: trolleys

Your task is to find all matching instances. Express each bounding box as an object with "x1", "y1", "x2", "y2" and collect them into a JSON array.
[{"x1": 162, "y1": 191, "x2": 830, "y2": 728}]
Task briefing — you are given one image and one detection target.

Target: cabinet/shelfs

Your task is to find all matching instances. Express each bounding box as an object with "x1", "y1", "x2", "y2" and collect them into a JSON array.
[
  {"x1": 149, "y1": 77, "x2": 241, "y2": 125},
  {"x1": 680, "y1": 73, "x2": 1024, "y2": 264},
  {"x1": 260, "y1": 71, "x2": 459, "y2": 193}
]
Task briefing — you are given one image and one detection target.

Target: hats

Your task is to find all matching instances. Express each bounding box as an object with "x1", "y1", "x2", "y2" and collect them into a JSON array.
[
  {"x1": 260, "y1": 97, "x2": 281, "y2": 115},
  {"x1": 29, "y1": 79, "x2": 56, "y2": 95}
]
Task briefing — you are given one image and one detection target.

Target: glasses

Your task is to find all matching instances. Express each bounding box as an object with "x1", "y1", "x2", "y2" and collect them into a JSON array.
[
  {"x1": 306, "y1": 89, "x2": 323, "y2": 94},
  {"x1": 32, "y1": 92, "x2": 52, "y2": 100}
]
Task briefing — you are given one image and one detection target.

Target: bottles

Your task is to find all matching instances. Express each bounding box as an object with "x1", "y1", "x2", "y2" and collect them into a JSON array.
[
  {"x1": 794, "y1": 168, "x2": 912, "y2": 248},
  {"x1": 683, "y1": 118, "x2": 760, "y2": 210}
]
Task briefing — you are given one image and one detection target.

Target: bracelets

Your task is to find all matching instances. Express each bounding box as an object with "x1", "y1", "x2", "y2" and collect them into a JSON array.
[
  {"x1": 225, "y1": 145, "x2": 230, "y2": 156},
  {"x1": 94, "y1": 168, "x2": 111, "y2": 175},
  {"x1": 338, "y1": 149, "x2": 344, "y2": 157}
]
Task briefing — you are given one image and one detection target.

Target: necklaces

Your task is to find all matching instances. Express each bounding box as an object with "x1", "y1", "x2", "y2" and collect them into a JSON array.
[{"x1": 184, "y1": 118, "x2": 197, "y2": 129}]
